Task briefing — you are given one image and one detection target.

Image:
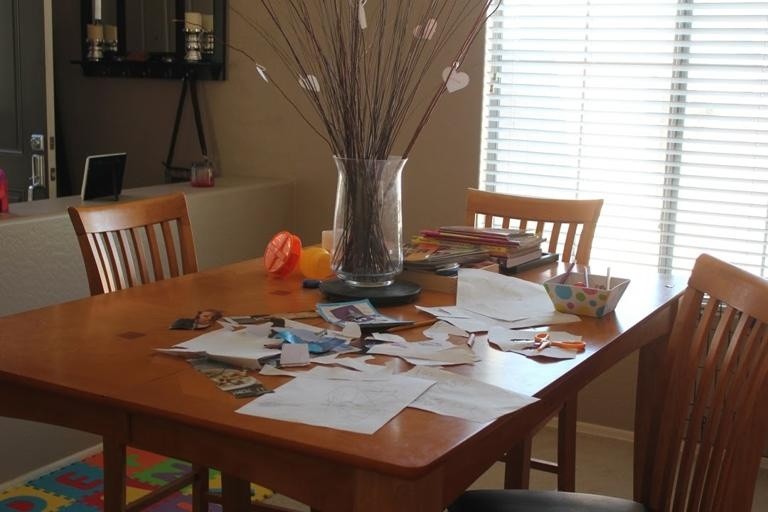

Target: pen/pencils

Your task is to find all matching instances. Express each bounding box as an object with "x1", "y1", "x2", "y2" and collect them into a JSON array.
[
  {"x1": 583, "y1": 266, "x2": 588, "y2": 288},
  {"x1": 466, "y1": 333, "x2": 475, "y2": 348},
  {"x1": 606, "y1": 267, "x2": 611, "y2": 290},
  {"x1": 265, "y1": 344, "x2": 281, "y2": 349},
  {"x1": 384, "y1": 318, "x2": 437, "y2": 333},
  {"x1": 560, "y1": 263, "x2": 575, "y2": 284}
]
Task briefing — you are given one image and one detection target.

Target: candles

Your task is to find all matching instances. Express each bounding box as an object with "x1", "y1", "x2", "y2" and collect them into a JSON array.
[
  {"x1": 200, "y1": 12, "x2": 214, "y2": 33},
  {"x1": 103, "y1": 24, "x2": 117, "y2": 42},
  {"x1": 86, "y1": 24, "x2": 103, "y2": 42},
  {"x1": 185, "y1": 12, "x2": 202, "y2": 33}
]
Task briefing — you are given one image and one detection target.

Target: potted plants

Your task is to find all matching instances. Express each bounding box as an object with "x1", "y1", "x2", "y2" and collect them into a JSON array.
[{"x1": 166, "y1": 0, "x2": 501, "y2": 288}]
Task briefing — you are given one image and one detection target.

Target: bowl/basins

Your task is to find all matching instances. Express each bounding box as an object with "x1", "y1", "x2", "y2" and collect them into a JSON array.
[{"x1": 543, "y1": 272, "x2": 631, "y2": 318}]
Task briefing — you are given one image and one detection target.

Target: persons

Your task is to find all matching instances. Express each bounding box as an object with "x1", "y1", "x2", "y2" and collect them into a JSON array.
[{"x1": 168, "y1": 308, "x2": 222, "y2": 331}]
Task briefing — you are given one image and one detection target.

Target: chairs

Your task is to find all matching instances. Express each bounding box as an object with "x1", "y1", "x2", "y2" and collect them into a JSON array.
[
  {"x1": 447, "y1": 251, "x2": 768, "y2": 511},
  {"x1": 65, "y1": 193, "x2": 256, "y2": 512},
  {"x1": 456, "y1": 186, "x2": 605, "y2": 490}
]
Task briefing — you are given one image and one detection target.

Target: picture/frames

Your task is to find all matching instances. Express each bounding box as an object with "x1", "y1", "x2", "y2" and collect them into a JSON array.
[{"x1": 80, "y1": 152, "x2": 127, "y2": 201}]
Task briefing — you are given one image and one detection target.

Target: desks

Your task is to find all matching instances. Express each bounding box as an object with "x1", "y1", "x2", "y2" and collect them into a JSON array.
[{"x1": 0, "y1": 231, "x2": 695, "y2": 512}]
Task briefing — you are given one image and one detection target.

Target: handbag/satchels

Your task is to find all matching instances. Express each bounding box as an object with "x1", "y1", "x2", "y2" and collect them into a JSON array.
[{"x1": 166, "y1": 164, "x2": 191, "y2": 183}]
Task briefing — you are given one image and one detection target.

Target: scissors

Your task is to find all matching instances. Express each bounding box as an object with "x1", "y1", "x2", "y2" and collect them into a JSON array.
[{"x1": 534, "y1": 333, "x2": 586, "y2": 352}]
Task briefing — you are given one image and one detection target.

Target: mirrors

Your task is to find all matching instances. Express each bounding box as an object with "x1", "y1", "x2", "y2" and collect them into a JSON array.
[{"x1": 80, "y1": 0, "x2": 227, "y2": 81}]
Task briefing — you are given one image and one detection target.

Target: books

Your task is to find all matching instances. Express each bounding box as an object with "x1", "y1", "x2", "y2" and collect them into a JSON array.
[{"x1": 400, "y1": 225, "x2": 550, "y2": 274}]
